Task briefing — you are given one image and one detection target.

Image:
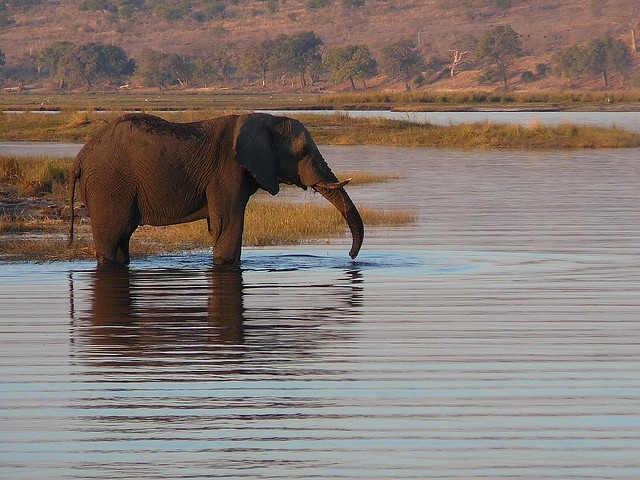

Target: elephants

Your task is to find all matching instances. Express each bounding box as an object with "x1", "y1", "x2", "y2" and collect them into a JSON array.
[{"x1": 65, "y1": 112, "x2": 364, "y2": 265}]
